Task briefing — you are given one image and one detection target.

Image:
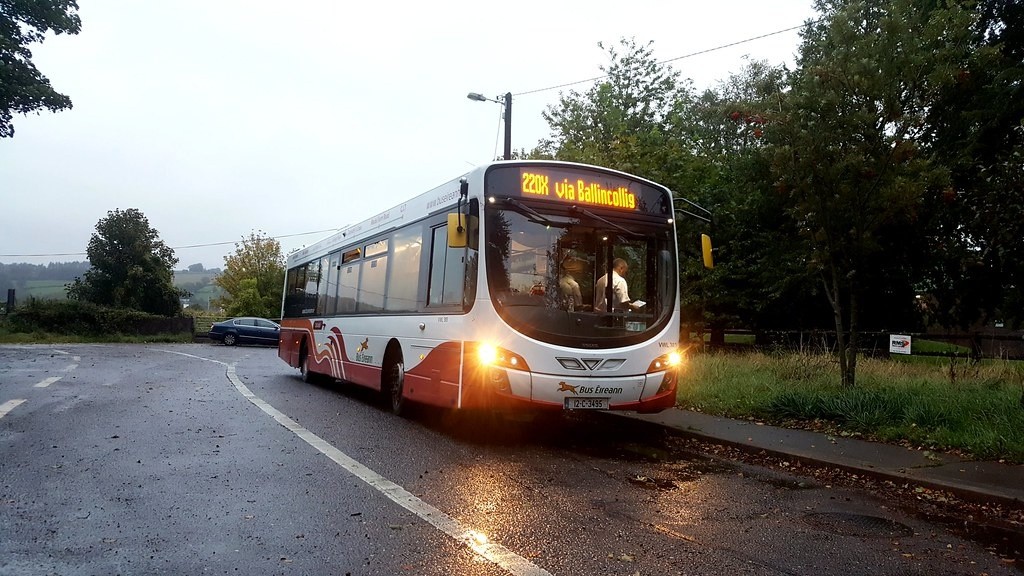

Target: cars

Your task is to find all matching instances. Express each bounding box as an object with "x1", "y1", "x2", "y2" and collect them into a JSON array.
[{"x1": 209, "y1": 317, "x2": 280, "y2": 346}]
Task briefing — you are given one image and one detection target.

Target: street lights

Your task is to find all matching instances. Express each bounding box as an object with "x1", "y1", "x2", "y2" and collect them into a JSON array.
[{"x1": 467, "y1": 91, "x2": 511, "y2": 160}]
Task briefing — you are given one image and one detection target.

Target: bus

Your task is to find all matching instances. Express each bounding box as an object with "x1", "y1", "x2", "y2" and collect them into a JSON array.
[{"x1": 279, "y1": 160, "x2": 717, "y2": 421}]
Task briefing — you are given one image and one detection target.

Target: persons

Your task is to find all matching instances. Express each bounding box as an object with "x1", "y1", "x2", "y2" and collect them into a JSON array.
[
  {"x1": 594, "y1": 258, "x2": 640, "y2": 314},
  {"x1": 558, "y1": 261, "x2": 583, "y2": 308}
]
